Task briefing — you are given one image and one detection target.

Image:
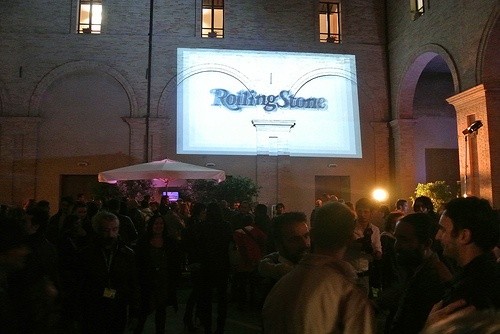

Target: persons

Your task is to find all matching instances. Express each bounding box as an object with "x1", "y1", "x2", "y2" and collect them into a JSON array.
[{"x1": 0, "y1": 189, "x2": 500, "y2": 334}]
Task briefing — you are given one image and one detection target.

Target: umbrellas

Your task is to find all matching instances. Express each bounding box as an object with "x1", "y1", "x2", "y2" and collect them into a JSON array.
[{"x1": 98, "y1": 156, "x2": 227, "y2": 196}]
